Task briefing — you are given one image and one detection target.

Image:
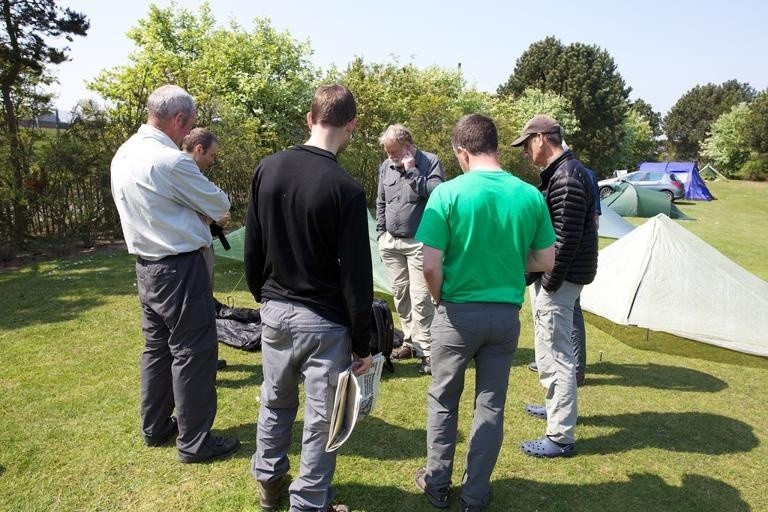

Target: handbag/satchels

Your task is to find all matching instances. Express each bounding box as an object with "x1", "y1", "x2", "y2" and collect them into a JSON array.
[{"x1": 367, "y1": 299, "x2": 394, "y2": 374}]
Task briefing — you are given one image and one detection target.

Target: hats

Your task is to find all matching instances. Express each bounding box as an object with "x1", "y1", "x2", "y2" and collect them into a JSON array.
[{"x1": 510, "y1": 114, "x2": 561, "y2": 148}]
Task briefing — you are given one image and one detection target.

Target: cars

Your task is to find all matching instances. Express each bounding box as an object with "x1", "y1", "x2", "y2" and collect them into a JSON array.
[{"x1": 595, "y1": 169, "x2": 685, "y2": 203}]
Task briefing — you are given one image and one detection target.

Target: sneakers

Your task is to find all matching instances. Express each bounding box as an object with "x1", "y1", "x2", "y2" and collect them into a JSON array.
[
  {"x1": 418, "y1": 356, "x2": 431, "y2": 375},
  {"x1": 391, "y1": 346, "x2": 416, "y2": 360},
  {"x1": 144, "y1": 416, "x2": 178, "y2": 447},
  {"x1": 414, "y1": 465, "x2": 451, "y2": 507},
  {"x1": 260, "y1": 474, "x2": 292, "y2": 511},
  {"x1": 459, "y1": 483, "x2": 492, "y2": 512},
  {"x1": 179, "y1": 436, "x2": 240, "y2": 463},
  {"x1": 216, "y1": 360, "x2": 226, "y2": 371}
]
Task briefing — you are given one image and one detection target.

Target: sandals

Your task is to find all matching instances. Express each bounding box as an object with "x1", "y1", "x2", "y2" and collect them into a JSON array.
[
  {"x1": 524, "y1": 405, "x2": 547, "y2": 419},
  {"x1": 520, "y1": 434, "x2": 576, "y2": 458}
]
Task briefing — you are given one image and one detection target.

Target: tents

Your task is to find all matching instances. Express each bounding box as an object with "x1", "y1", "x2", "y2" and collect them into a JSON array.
[{"x1": 579, "y1": 161, "x2": 768, "y2": 356}]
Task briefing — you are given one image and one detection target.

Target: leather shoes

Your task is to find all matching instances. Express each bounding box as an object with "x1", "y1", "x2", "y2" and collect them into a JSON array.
[{"x1": 527, "y1": 362, "x2": 538, "y2": 372}]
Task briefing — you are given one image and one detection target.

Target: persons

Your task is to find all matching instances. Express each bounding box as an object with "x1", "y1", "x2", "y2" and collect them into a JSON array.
[
  {"x1": 244, "y1": 84, "x2": 374, "y2": 512},
  {"x1": 376, "y1": 124, "x2": 445, "y2": 375},
  {"x1": 181, "y1": 127, "x2": 232, "y2": 370},
  {"x1": 109, "y1": 84, "x2": 240, "y2": 462},
  {"x1": 416, "y1": 113, "x2": 557, "y2": 512},
  {"x1": 528, "y1": 125, "x2": 603, "y2": 385},
  {"x1": 510, "y1": 115, "x2": 598, "y2": 457}
]
